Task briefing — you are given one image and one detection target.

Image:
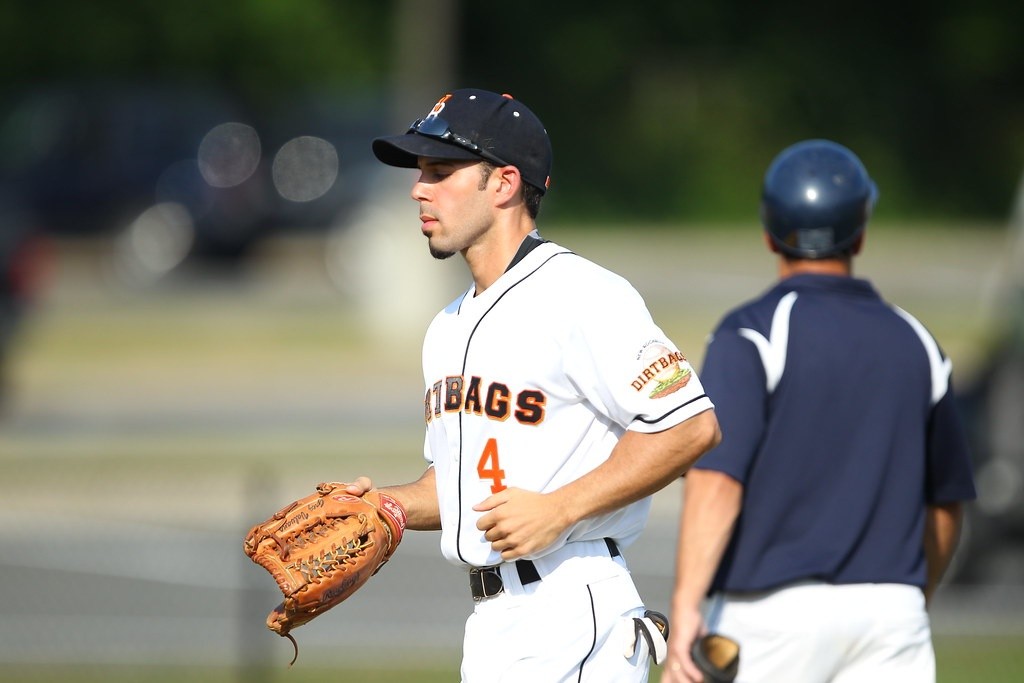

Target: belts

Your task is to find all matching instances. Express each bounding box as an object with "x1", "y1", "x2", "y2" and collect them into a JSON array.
[{"x1": 471, "y1": 537, "x2": 622, "y2": 602}]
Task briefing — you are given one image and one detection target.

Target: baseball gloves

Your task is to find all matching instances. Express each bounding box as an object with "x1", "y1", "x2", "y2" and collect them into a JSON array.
[{"x1": 242, "y1": 481, "x2": 409, "y2": 669}]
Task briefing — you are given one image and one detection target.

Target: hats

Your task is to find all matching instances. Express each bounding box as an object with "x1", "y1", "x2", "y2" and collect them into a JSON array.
[{"x1": 372, "y1": 88, "x2": 552, "y2": 193}]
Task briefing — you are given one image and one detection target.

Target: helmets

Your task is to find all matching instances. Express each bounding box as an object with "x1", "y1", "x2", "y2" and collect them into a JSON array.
[{"x1": 759, "y1": 136, "x2": 873, "y2": 261}]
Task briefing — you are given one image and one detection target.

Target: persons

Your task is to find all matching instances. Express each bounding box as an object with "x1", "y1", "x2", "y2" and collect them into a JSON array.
[
  {"x1": 657, "y1": 137, "x2": 978, "y2": 682},
  {"x1": 321, "y1": 88, "x2": 721, "y2": 683}
]
